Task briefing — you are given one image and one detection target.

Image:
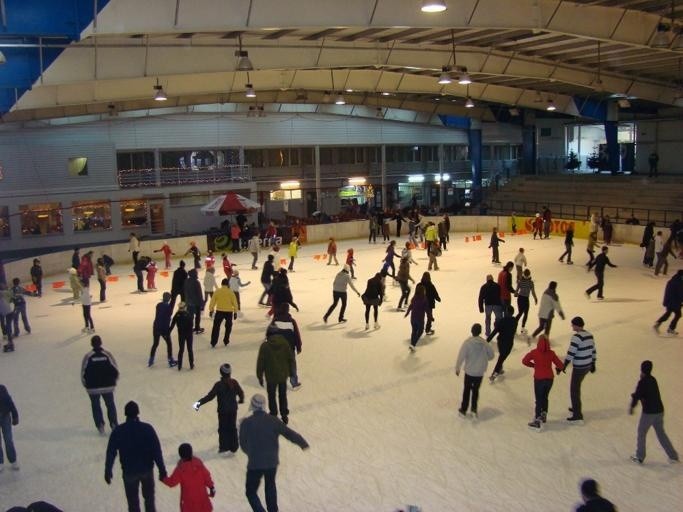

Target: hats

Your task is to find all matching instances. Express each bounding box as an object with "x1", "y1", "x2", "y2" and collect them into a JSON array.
[
  {"x1": 571, "y1": 317, "x2": 583, "y2": 327},
  {"x1": 251, "y1": 394, "x2": 265, "y2": 411},
  {"x1": 266, "y1": 321, "x2": 277, "y2": 338},
  {"x1": 179, "y1": 444, "x2": 191, "y2": 458},
  {"x1": 219, "y1": 364, "x2": 230, "y2": 377},
  {"x1": 177, "y1": 302, "x2": 188, "y2": 312},
  {"x1": 470, "y1": 324, "x2": 481, "y2": 334},
  {"x1": 124, "y1": 401, "x2": 138, "y2": 416}
]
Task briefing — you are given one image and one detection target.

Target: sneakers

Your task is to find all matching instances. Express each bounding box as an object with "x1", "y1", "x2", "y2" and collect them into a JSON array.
[
  {"x1": 566, "y1": 408, "x2": 582, "y2": 420},
  {"x1": 527, "y1": 412, "x2": 545, "y2": 428}
]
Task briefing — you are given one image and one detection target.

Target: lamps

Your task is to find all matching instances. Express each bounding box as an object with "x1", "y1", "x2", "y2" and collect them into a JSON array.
[
  {"x1": 236, "y1": 56, "x2": 253, "y2": 70},
  {"x1": 456, "y1": 64, "x2": 472, "y2": 85},
  {"x1": 246, "y1": 85, "x2": 256, "y2": 98},
  {"x1": 154, "y1": 87, "x2": 167, "y2": 102},
  {"x1": 335, "y1": 91, "x2": 346, "y2": 106},
  {"x1": 437, "y1": 64, "x2": 452, "y2": 84},
  {"x1": 649, "y1": 14, "x2": 671, "y2": 49},
  {"x1": 609, "y1": 91, "x2": 628, "y2": 100},
  {"x1": 464, "y1": 85, "x2": 475, "y2": 108}
]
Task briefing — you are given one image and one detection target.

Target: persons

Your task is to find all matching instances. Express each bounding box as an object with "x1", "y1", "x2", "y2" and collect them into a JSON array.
[
  {"x1": 649, "y1": 149, "x2": 659, "y2": 178},
  {"x1": 455, "y1": 249, "x2": 683, "y2": 512},
  {"x1": 323, "y1": 205, "x2": 451, "y2": 353},
  {"x1": 1, "y1": 215, "x2": 310, "y2": 511},
  {"x1": 533, "y1": 206, "x2": 683, "y2": 300},
  {"x1": 489, "y1": 227, "x2": 505, "y2": 264}
]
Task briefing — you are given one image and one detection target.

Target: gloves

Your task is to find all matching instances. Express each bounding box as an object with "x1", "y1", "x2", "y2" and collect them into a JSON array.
[
  {"x1": 210, "y1": 486, "x2": 216, "y2": 498},
  {"x1": 193, "y1": 402, "x2": 201, "y2": 410}
]
